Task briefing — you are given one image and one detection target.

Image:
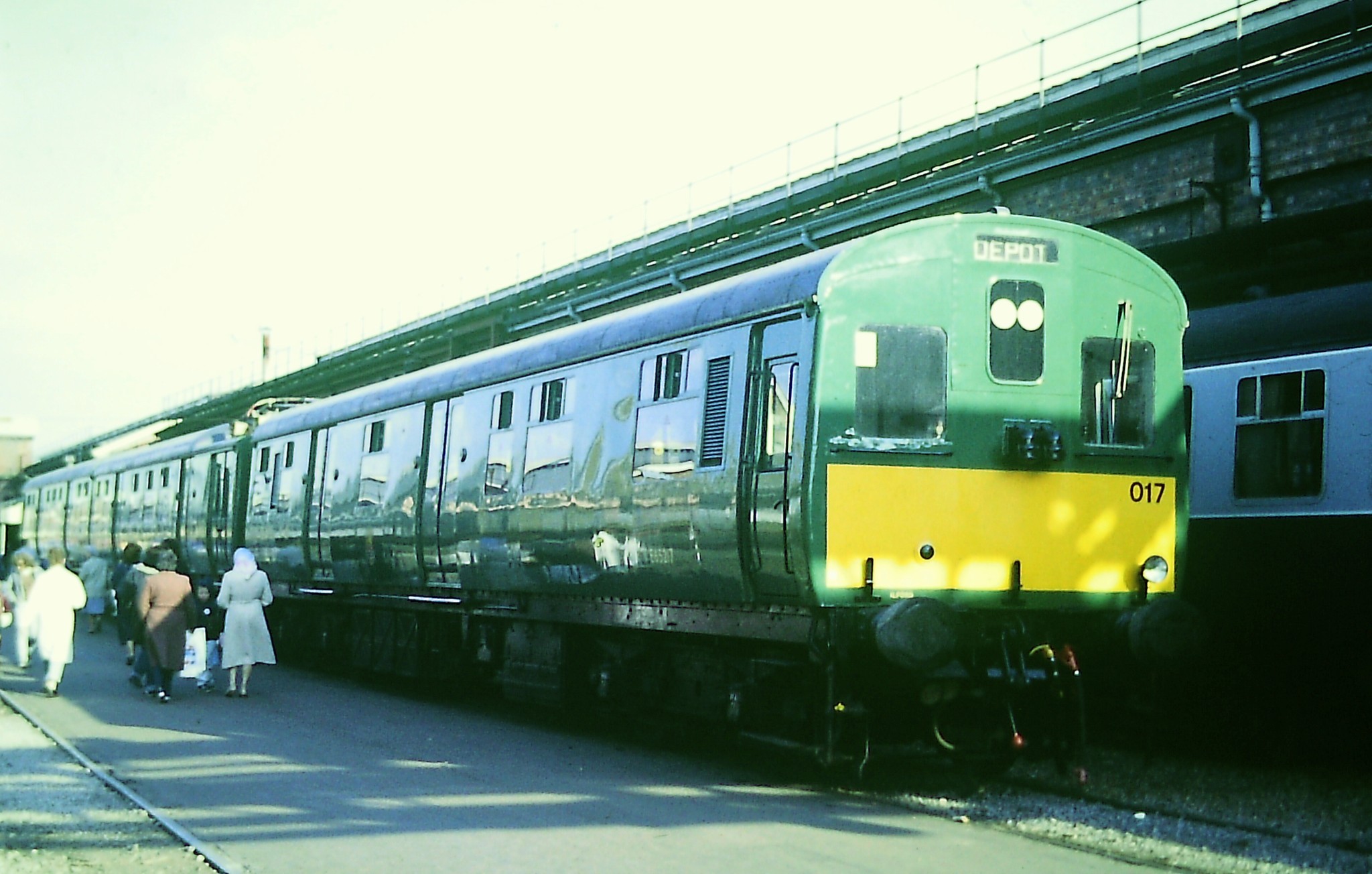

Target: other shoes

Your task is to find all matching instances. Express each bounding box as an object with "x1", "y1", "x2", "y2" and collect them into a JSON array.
[
  {"x1": 126, "y1": 655, "x2": 136, "y2": 665},
  {"x1": 157, "y1": 690, "x2": 171, "y2": 703},
  {"x1": 224, "y1": 685, "x2": 248, "y2": 697},
  {"x1": 35, "y1": 687, "x2": 59, "y2": 699}
]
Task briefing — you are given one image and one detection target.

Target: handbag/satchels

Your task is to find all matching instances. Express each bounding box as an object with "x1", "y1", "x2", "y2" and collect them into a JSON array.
[{"x1": 179, "y1": 627, "x2": 207, "y2": 679}]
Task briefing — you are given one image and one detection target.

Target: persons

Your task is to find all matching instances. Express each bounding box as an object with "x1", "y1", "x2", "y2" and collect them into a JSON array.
[
  {"x1": 216, "y1": 547, "x2": 276, "y2": 698},
  {"x1": 27, "y1": 547, "x2": 87, "y2": 697},
  {"x1": 136, "y1": 549, "x2": 198, "y2": 703},
  {"x1": 0, "y1": 538, "x2": 224, "y2": 693}
]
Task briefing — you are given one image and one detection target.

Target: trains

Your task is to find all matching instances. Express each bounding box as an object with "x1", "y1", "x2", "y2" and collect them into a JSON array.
[{"x1": 18, "y1": 206, "x2": 1196, "y2": 799}]
